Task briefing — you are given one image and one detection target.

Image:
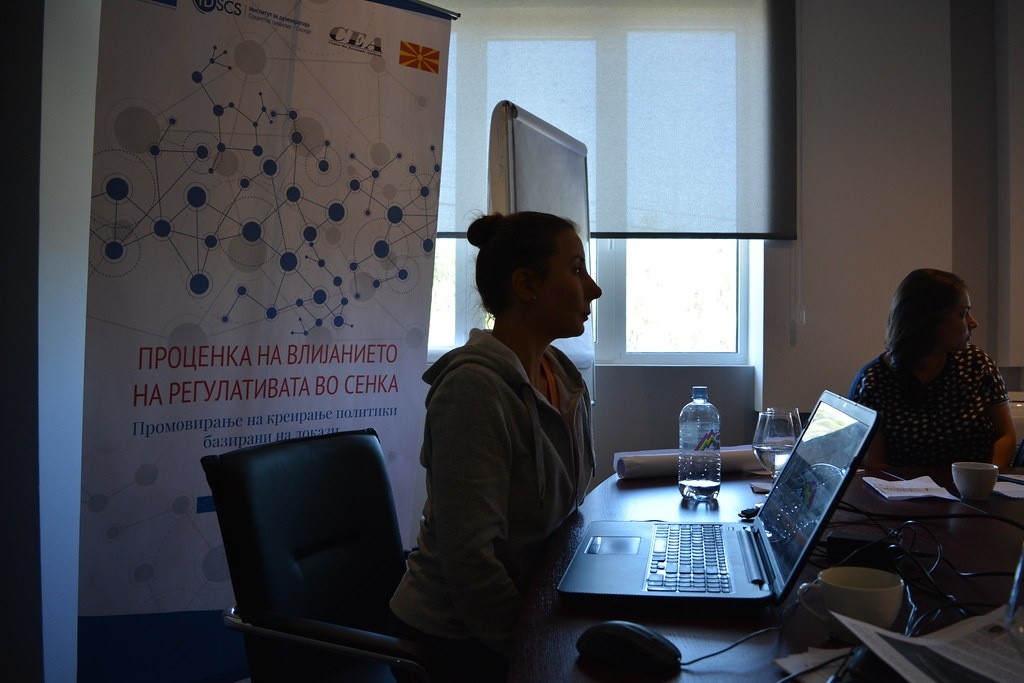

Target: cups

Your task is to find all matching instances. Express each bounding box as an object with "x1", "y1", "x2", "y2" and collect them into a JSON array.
[
  {"x1": 798, "y1": 566, "x2": 904, "y2": 645},
  {"x1": 952, "y1": 462, "x2": 999, "y2": 501},
  {"x1": 763, "y1": 408, "x2": 803, "y2": 498}
]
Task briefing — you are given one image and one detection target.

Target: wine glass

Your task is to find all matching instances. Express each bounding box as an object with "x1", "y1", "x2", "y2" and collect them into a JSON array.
[{"x1": 752, "y1": 412, "x2": 795, "y2": 509}]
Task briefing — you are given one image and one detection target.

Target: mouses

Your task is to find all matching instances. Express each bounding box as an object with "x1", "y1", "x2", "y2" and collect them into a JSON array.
[{"x1": 575, "y1": 620, "x2": 682, "y2": 671}]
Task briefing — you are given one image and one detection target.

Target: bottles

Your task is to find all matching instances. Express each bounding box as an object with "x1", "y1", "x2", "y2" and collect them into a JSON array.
[{"x1": 678, "y1": 386, "x2": 721, "y2": 501}]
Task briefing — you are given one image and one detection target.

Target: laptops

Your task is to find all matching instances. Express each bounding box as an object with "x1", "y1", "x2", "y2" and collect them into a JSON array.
[{"x1": 556, "y1": 390, "x2": 881, "y2": 612}]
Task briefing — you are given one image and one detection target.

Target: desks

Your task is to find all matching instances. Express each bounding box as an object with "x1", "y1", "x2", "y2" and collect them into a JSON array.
[{"x1": 508, "y1": 443, "x2": 1024, "y2": 683}]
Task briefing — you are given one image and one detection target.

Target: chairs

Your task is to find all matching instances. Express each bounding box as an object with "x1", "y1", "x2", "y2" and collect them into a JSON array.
[{"x1": 199, "y1": 427, "x2": 449, "y2": 683}]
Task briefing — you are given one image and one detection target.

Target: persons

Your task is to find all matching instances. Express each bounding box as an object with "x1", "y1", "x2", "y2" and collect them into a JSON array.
[
  {"x1": 849, "y1": 268, "x2": 1017, "y2": 468},
  {"x1": 390, "y1": 210, "x2": 602, "y2": 683},
  {"x1": 766, "y1": 463, "x2": 843, "y2": 571}
]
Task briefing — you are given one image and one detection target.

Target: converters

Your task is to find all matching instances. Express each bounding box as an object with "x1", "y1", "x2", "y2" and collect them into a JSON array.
[{"x1": 826, "y1": 530, "x2": 904, "y2": 566}]
